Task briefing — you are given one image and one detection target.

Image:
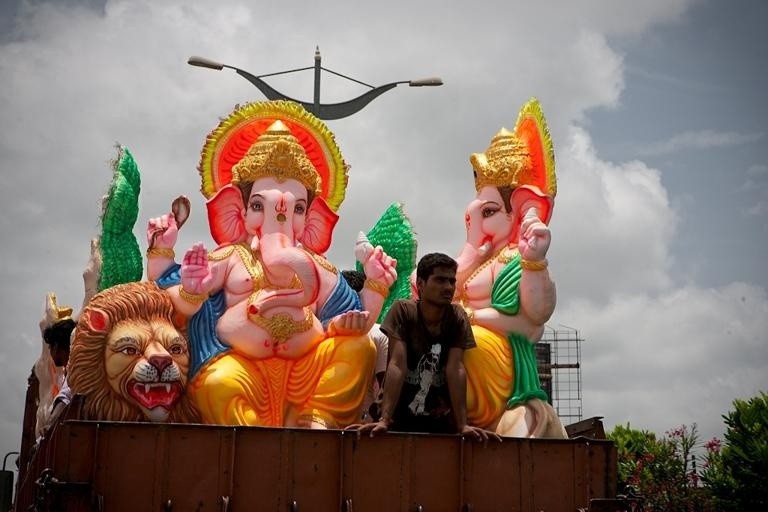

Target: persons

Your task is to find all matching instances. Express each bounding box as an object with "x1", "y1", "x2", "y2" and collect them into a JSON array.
[{"x1": 341, "y1": 249, "x2": 506, "y2": 445}]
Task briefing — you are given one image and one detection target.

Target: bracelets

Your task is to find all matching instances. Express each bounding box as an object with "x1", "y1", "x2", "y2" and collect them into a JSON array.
[{"x1": 379, "y1": 414, "x2": 393, "y2": 424}]
